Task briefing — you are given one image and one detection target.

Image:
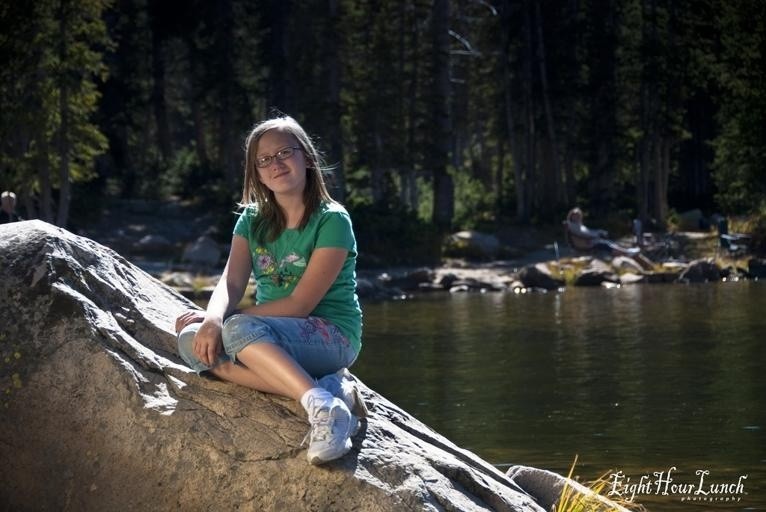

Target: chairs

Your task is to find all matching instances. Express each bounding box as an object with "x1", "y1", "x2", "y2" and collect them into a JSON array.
[{"x1": 567, "y1": 231, "x2": 594, "y2": 256}]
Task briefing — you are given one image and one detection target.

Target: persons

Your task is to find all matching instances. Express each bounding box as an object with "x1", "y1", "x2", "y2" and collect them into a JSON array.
[
  {"x1": 0, "y1": 188, "x2": 23, "y2": 227},
  {"x1": 564, "y1": 204, "x2": 643, "y2": 259},
  {"x1": 173, "y1": 110, "x2": 366, "y2": 468}
]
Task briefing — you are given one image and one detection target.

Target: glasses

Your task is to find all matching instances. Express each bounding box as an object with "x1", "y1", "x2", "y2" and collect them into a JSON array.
[{"x1": 253, "y1": 146, "x2": 303, "y2": 169}]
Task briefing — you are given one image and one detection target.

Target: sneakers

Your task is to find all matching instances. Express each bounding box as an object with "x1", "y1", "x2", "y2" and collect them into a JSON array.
[
  {"x1": 315, "y1": 367, "x2": 368, "y2": 420},
  {"x1": 304, "y1": 399, "x2": 360, "y2": 465}
]
